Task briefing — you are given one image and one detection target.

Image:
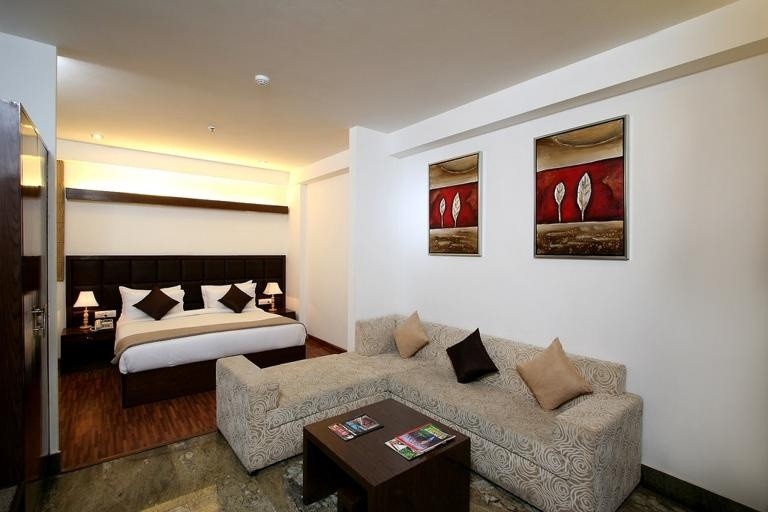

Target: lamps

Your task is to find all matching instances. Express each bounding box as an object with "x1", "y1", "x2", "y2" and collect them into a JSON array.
[
  {"x1": 73, "y1": 291, "x2": 100, "y2": 330},
  {"x1": 263, "y1": 282, "x2": 283, "y2": 311}
]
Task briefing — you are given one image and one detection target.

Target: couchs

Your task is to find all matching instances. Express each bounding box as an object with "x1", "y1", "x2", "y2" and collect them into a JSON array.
[{"x1": 216, "y1": 314, "x2": 643, "y2": 512}]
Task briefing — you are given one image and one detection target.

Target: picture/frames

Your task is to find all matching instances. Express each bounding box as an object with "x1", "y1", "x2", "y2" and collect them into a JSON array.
[
  {"x1": 428, "y1": 151, "x2": 483, "y2": 257},
  {"x1": 534, "y1": 114, "x2": 628, "y2": 261}
]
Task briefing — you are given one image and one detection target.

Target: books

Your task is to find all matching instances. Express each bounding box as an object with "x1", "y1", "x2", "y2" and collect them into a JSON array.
[
  {"x1": 384, "y1": 422, "x2": 456, "y2": 461},
  {"x1": 328, "y1": 413, "x2": 380, "y2": 441}
]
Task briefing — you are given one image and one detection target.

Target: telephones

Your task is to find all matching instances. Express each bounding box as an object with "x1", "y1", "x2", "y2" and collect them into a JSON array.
[{"x1": 94, "y1": 319, "x2": 114, "y2": 331}]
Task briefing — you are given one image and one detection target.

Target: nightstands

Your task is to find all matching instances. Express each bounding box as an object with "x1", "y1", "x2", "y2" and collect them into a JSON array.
[
  {"x1": 61, "y1": 327, "x2": 116, "y2": 371},
  {"x1": 264, "y1": 307, "x2": 296, "y2": 320}
]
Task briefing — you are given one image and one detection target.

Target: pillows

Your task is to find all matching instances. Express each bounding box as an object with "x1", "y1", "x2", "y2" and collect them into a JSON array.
[
  {"x1": 119, "y1": 280, "x2": 257, "y2": 322},
  {"x1": 516, "y1": 337, "x2": 592, "y2": 411},
  {"x1": 446, "y1": 328, "x2": 498, "y2": 383},
  {"x1": 392, "y1": 310, "x2": 429, "y2": 358}
]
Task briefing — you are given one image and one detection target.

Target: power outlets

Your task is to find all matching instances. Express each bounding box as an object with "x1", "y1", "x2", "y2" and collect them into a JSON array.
[
  {"x1": 95, "y1": 310, "x2": 116, "y2": 319},
  {"x1": 258, "y1": 298, "x2": 272, "y2": 305}
]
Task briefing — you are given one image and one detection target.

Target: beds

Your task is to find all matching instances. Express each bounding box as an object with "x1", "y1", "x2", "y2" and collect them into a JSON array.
[{"x1": 122, "y1": 309, "x2": 306, "y2": 408}]
